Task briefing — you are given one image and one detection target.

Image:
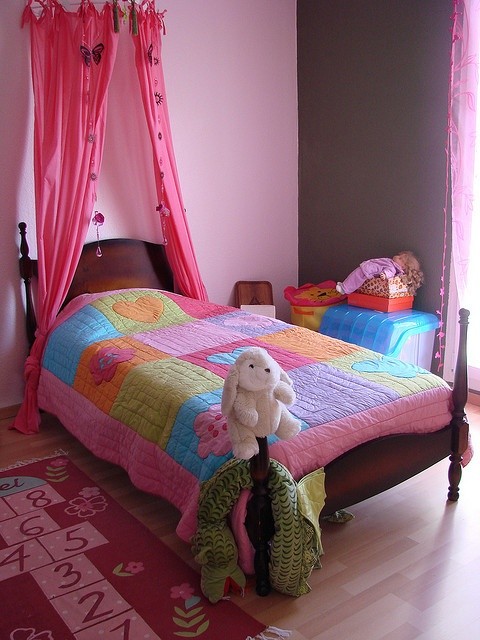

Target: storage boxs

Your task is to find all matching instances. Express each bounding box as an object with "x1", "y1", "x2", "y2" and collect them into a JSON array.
[
  {"x1": 346, "y1": 291, "x2": 414, "y2": 312},
  {"x1": 318, "y1": 303, "x2": 440, "y2": 372},
  {"x1": 358, "y1": 274, "x2": 414, "y2": 298}
]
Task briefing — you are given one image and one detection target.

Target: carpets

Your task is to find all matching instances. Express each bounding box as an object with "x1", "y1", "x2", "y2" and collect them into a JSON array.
[{"x1": 1, "y1": 449, "x2": 291, "y2": 639}]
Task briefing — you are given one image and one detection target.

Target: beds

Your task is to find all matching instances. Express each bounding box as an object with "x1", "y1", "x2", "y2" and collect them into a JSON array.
[{"x1": 19, "y1": 221, "x2": 470, "y2": 597}]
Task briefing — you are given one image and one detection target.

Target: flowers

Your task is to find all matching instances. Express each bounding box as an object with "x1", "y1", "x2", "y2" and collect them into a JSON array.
[
  {"x1": 45, "y1": 458, "x2": 69, "y2": 482},
  {"x1": 194, "y1": 404, "x2": 232, "y2": 458},
  {"x1": 64, "y1": 496, "x2": 108, "y2": 517},
  {"x1": 89, "y1": 347, "x2": 137, "y2": 386},
  {"x1": 92, "y1": 211, "x2": 104, "y2": 226},
  {"x1": 78, "y1": 487, "x2": 100, "y2": 498},
  {"x1": 170, "y1": 583, "x2": 210, "y2": 638},
  {"x1": 107, "y1": 561, "x2": 144, "y2": 579}
]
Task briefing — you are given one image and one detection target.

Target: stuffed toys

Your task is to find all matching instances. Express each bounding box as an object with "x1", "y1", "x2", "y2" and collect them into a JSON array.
[
  {"x1": 220, "y1": 347, "x2": 302, "y2": 460},
  {"x1": 191, "y1": 458, "x2": 353, "y2": 603}
]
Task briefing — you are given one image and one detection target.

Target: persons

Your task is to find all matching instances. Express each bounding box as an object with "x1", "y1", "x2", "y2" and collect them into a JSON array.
[{"x1": 336, "y1": 251, "x2": 424, "y2": 296}]
